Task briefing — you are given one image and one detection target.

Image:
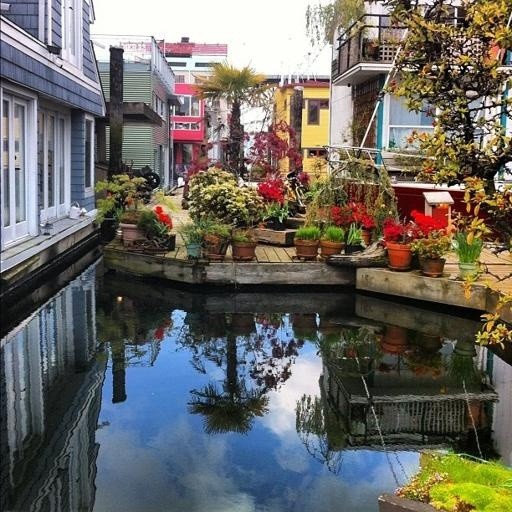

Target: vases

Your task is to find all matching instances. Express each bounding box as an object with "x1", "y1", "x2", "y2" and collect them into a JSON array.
[
  {"x1": 387, "y1": 243, "x2": 411, "y2": 271},
  {"x1": 352, "y1": 229, "x2": 371, "y2": 246},
  {"x1": 382, "y1": 326, "x2": 409, "y2": 353}
]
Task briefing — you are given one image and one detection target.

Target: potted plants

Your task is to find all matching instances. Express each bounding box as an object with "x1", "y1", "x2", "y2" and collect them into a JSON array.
[
  {"x1": 319, "y1": 318, "x2": 342, "y2": 343},
  {"x1": 412, "y1": 231, "x2": 450, "y2": 277},
  {"x1": 292, "y1": 227, "x2": 318, "y2": 259},
  {"x1": 232, "y1": 230, "x2": 258, "y2": 260},
  {"x1": 410, "y1": 334, "x2": 444, "y2": 372},
  {"x1": 321, "y1": 226, "x2": 345, "y2": 258},
  {"x1": 449, "y1": 341, "x2": 478, "y2": 387},
  {"x1": 206, "y1": 224, "x2": 231, "y2": 260},
  {"x1": 177, "y1": 223, "x2": 204, "y2": 258},
  {"x1": 293, "y1": 313, "x2": 318, "y2": 341},
  {"x1": 119, "y1": 212, "x2": 148, "y2": 246},
  {"x1": 453, "y1": 230, "x2": 484, "y2": 281},
  {"x1": 232, "y1": 314, "x2": 255, "y2": 338},
  {"x1": 261, "y1": 205, "x2": 288, "y2": 231}
]
